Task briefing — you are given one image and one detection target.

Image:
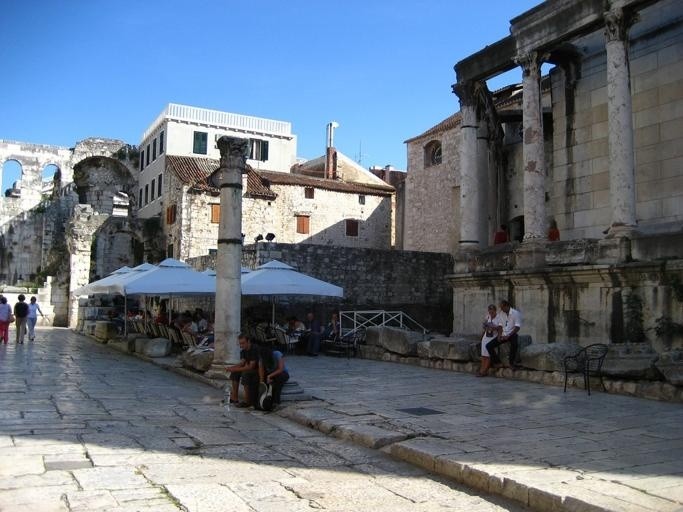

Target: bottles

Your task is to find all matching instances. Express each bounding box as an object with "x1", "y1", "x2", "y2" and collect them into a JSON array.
[{"x1": 223, "y1": 384, "x2": 230, "y2": 405}]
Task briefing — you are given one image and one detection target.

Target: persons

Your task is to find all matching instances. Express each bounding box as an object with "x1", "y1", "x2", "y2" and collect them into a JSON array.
[
  {"x1": 492, "y1": 224, "x2": 510, "y2": 246},
  {"x1": 0, "y1": 296, "x2": 11, "y2": 344},
  {"x1": 547, "y1": 219, "x2": 560, "y2": 242},
  {"x1": 13, "y1": 294, "x2": 28, "y2": 344},
  {"x1": 252, "y1": 346, "x2": 289, "y2": 409},
  {"x1": 484, "y1": 299, "x2": 523, "y2": 373},
  {"x1": 26, "y1": 296, "x2": 43, "y2": 341},
  {"x1": 105, "y1": 305, "x2": 340, "y2": 357},
  {"x1": 474, "y1": 304, "x2": 498, "y2": 377},
  {"x1": 225, "y1": 334, "x2": 257, "y2": 408}
]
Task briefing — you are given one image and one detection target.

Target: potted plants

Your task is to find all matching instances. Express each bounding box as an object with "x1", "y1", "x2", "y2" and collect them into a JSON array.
[{"x1": 549, "y1": 220, "x2": 559, "y2": 240}]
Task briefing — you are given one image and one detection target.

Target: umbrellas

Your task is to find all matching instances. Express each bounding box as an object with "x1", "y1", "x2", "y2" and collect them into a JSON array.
[{"x1": 71, "y1": 255, "x2": 344, "y2": 307}]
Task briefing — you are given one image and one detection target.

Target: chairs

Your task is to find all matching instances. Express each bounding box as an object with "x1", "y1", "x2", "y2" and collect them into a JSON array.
[
  {"x1": 130, "y1": 319, "x2": 362, "y2": 359},
  {"x1": 563, "y1": 343, "x2": 607, "y2": 395}
]
